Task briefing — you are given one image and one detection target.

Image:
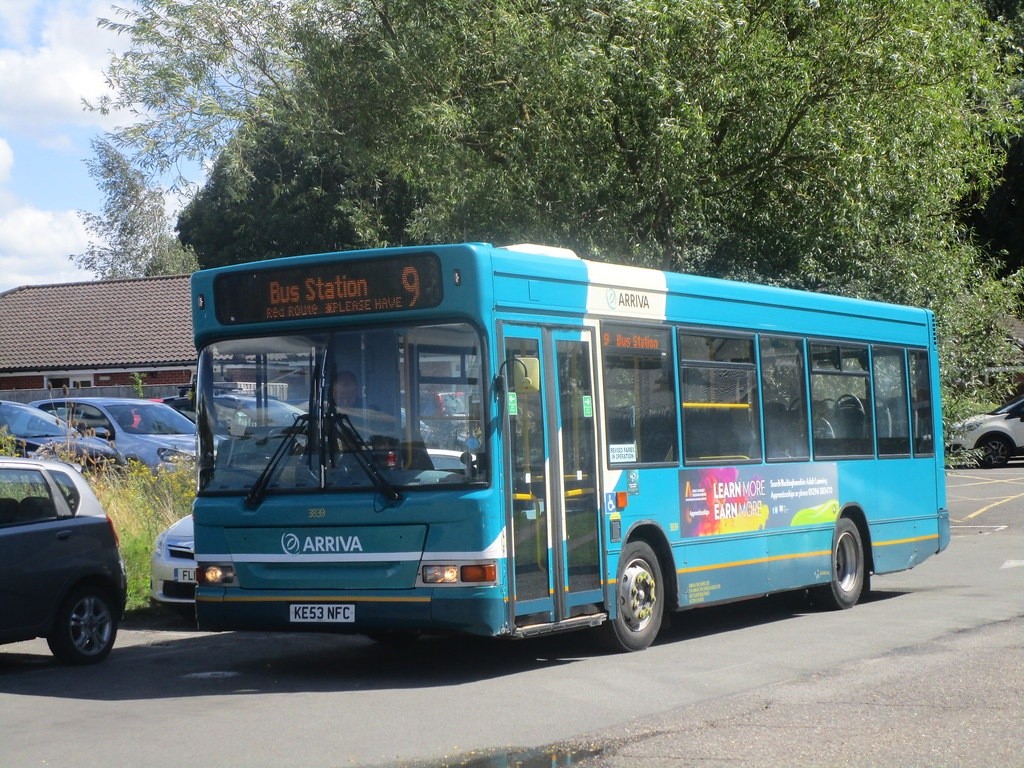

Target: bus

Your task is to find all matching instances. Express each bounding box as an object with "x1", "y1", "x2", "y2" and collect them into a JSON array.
[{"x1": 190, "y1": 243, "x2": 950, "y2": 664}]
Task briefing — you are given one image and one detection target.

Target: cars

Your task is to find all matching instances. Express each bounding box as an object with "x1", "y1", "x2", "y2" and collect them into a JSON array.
[
  {"x1": 9, "y1": 397, "x2": 228, "y2": 475},
  {"x1": 295, "y1": 392, "x2": 478, "y2": 470},
  {"x1": 946, "y1": 395, "x2": 1024, "y2": 470},
  {"x1": 0, "y1": 456, "x2": 129, "y2": 669},
  {"x1": 165, "y1": 394, "x2": 307, "y2": 438},
  {"x1": 147, "y1": 512, "x2": 201, "y2": 605},
  {"x1": 0, "y1": 400, "x2": 128, "y2": 476}
]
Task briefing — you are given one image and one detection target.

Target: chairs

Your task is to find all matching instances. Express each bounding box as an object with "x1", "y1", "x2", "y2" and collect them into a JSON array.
[
  {"x1": 120, "y1": 411, "x2": 143, "y2": 434},
  {"x1": 1, "y1": 495, "x2": 60, "y2": 527},
  {"x1": 764, "y1": 394, "x2": 921, "y2": 452}
]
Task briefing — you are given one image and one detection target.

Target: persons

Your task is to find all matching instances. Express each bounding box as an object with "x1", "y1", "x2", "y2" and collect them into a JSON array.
[{"x1": 284, "y1": 370, "x2": 396, "y2": 451}]
